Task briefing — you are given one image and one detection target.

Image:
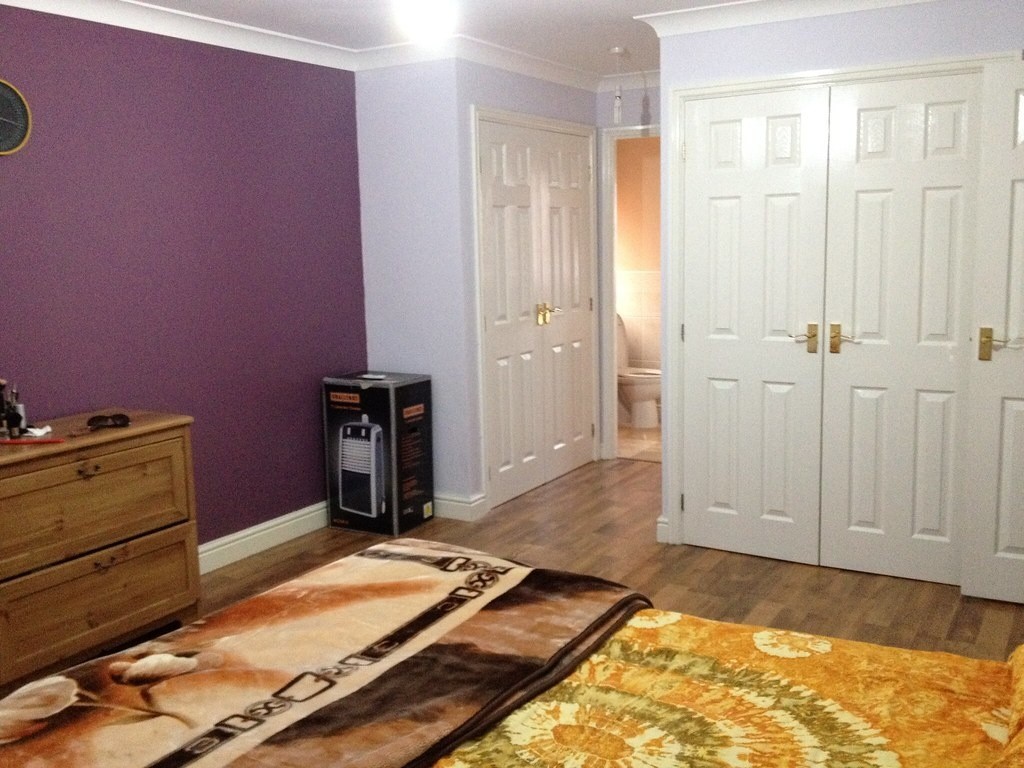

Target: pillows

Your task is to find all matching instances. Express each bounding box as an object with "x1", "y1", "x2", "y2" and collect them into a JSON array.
[
  {"x1": 986, "y1": 725, "x2": 1024, "y2": 767},
  {"x1": 1008, "y1": 642, "x2": 1023, "y2": 740}
]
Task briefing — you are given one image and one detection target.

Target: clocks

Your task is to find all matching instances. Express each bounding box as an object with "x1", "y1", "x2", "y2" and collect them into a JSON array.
[{"x1": 0, "y1": 78, "x2": 33, "y2": 155}]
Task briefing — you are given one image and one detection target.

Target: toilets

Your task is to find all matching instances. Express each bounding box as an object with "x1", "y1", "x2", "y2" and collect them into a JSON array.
[{"x1": 616, "y1": 313, "x2": 662, "y2": 428}]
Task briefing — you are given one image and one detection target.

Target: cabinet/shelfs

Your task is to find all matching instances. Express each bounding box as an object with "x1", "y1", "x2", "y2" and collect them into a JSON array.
[{"x1": 0, "y1": 405, "x2": 204, "y2": 706}]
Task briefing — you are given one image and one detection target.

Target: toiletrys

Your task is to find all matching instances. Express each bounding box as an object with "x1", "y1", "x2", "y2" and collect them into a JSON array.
[{"x1": 0, "y1": 379, "x2": 26, "y2": 439}]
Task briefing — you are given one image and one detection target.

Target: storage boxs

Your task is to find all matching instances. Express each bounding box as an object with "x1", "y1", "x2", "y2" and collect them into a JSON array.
[{"x1": 321, "y1": 371, "x2": 437, "y2": 537}]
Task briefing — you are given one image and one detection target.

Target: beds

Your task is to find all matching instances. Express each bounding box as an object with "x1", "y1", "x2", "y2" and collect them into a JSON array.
[{"x1": 0, "y1": 537, "x2": 1024, "y2": 767}]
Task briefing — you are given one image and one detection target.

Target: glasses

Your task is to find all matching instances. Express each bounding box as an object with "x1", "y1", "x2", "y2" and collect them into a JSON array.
[{"x1": 87, "y1": 415, "x2": 131, "y2": 431}]
